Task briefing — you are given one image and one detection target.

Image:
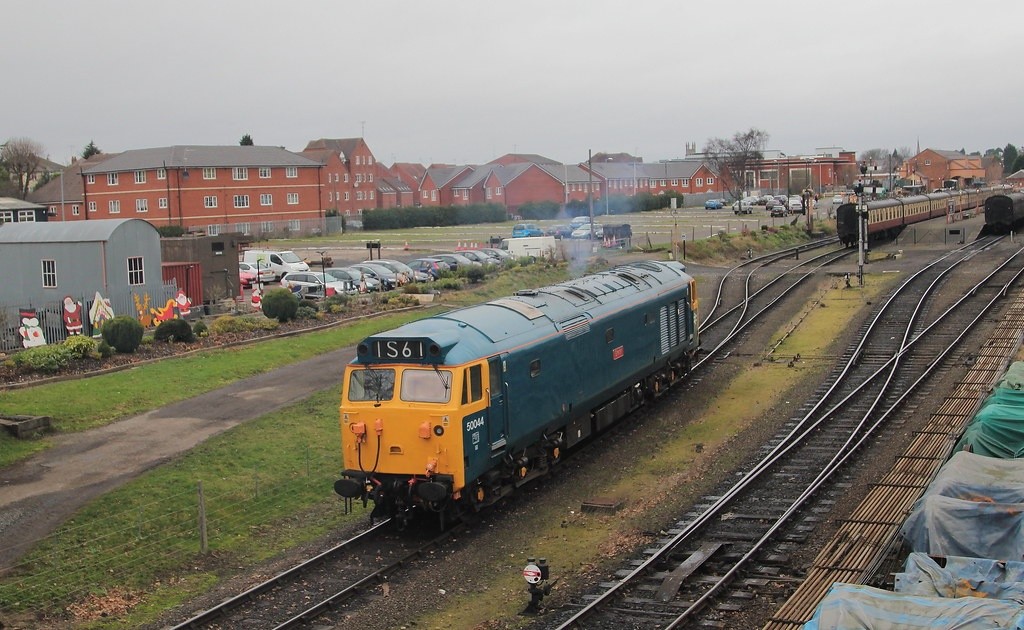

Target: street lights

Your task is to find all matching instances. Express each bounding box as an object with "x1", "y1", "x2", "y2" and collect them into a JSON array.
[
  {"x1": 779, "y1": 152, "x2": 790, "y2": 198},
  {"x1": 604, "y1": 157, "x2": 614, "y2": 217}
]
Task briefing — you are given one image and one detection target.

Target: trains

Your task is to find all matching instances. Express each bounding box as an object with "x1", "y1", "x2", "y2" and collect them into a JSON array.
[
  {"x1": 333, "y1": 258, "x2": 705, "y2": 530},
  {"x1": 984, "y1": 191, "x2": 1024, "y2": 236},
  {"x1": 836, "y1": 184, "x2": 1014, "y2": 248}
]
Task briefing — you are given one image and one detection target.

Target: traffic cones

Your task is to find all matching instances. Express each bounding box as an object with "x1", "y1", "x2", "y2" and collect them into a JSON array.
[
  {"x1": 456, "y1": 239, "x2": 478, "y2": 250},
  {"x1": 403, "y1": 239, "x2": 411, "y2": 248}
]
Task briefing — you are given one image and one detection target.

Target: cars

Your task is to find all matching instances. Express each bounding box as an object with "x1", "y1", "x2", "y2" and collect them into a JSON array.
[
  {"x1": 570, "y1": 216, "x2": 598, "y2": 228},
  {"x1": 546, "y1": 224, "x2": 604, "y2": 239},
  {"x1": 238, "y1": 262, "x2": 276, "y2": 285},
  {"x1": 704, "y1": 198, "x2": 727, "y2": 210},
  {"x1": 732, "y1": 194, "x2": 817, "y2": 218},
  {"x1": 277, "y1": 247, "x2": 517, "y2": 299},
  {"x1": 832, "y1": 195, "x2": 843, "y2": 204},
  {"x1": 239, "y1": 268, "x2": 259, "y2": 289},
  {"x1": 511, "y1": 224, "x2": 545, "y2": 238},
  {"x1": 268, "y1": 250, "x2": 310, "y2": 278}
]
